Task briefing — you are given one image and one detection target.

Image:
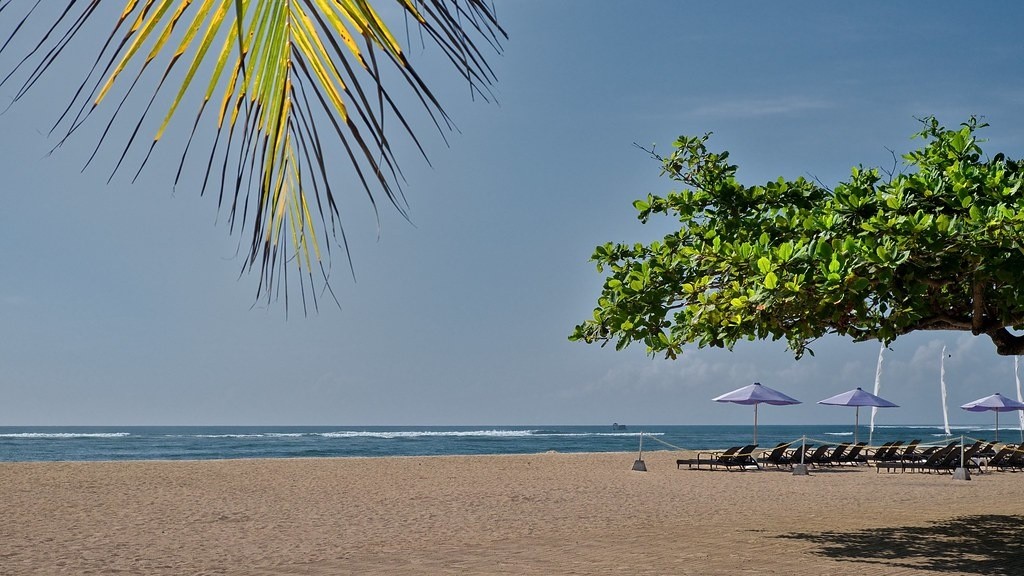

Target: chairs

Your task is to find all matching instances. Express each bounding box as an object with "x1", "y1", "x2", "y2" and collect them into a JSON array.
[
  {"x1": 691, "y1": 443, "x2": 762, "y2": 471},
  {"x1": 677, "y1": 445, "x2": 744, "y2": 471},
  {"x1": 757, "y1": 439, "x2": 1024, "y2": 475}
]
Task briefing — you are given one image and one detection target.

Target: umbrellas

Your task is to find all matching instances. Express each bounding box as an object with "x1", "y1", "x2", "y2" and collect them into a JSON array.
[
  {"x1": 712, "y1": 382, "x2": 801, "y2": 445},
  {"x1": 817, "y1": 387, "x2": 901, "y2": 444},
  {"x1": 961, "y1": 393, "x2": 1024, "y2": 442}
]
